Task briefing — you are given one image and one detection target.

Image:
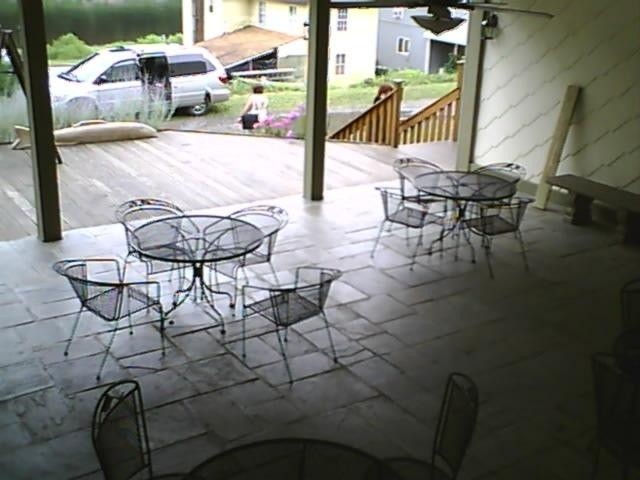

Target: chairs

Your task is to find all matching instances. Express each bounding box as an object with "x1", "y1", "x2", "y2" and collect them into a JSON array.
[
  {"x1": 382, "y1": 372, "x2": 479, "y2": 479},
  {"x1": 242, "y1": 265, "x2": 344, "y2": 381},
  {"x1": 53, "y1": 259, "x2": 167, "y2": 380},
  {"x1": 203, "y1": 206, "x2": 289, "y2": 308},
  {"x1": 91, "y1": 379, "x2": 185, "y2": 479},
  {"x1": 455, "y1": 197, "x2": 535, "y2": 279},
  {"x1": 116, "y1": 198, "x2": 200, "y2": 306},
  {"x1": 451, "y1": 162, "x2": 525, "y2": 247},
  {"x1": 388, "y1": 157, "x2": 457, "y2": 246},
  {"x1": 371, "y1": 187, "x2": 447, "y2": 272}
]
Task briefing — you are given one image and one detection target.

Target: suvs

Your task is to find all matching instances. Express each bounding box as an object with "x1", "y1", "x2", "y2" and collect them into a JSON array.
[{"x1": 49, "y1": 42, "x2": 231, "y2": 127}]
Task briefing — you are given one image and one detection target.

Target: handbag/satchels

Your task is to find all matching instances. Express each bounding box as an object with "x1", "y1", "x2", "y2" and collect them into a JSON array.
[{"x1": 242, "y1": 114, "x2": 258, "y2": 129}]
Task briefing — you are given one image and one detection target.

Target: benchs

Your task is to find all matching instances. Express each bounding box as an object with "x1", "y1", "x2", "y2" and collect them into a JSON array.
[{"x1": 546, "y1": 174, "x2": 640, "y2": 244}]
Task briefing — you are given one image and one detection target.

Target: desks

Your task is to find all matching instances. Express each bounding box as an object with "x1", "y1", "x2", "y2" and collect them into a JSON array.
[
  {"x1": 179, "y1": 438, "x2": 403, "y2": 480},
  {"x1": 129, "y1": 216, "x2": 264, "y2": 334},
  {"x1": 414, "y1": 171, "x2": 517, "y2": 257}
]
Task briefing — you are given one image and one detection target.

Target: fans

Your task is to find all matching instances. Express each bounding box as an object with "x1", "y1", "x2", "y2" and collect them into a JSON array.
[{"x1": 327, "y1": 1, "x2": 555, "y2": 34}]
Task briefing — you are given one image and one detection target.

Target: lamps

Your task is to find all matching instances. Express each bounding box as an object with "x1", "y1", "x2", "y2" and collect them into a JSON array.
[{"x1": 482, "y1": 14, "x2": 498, "y2": 40}]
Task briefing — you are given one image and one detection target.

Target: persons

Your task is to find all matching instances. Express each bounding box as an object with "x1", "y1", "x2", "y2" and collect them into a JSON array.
[
  {"x1": 374, "y1": 84, "x2": 393, "y2": 104},
  {"x1": 237, "y1": 84, "x2": 269, "y2": 134}
]
